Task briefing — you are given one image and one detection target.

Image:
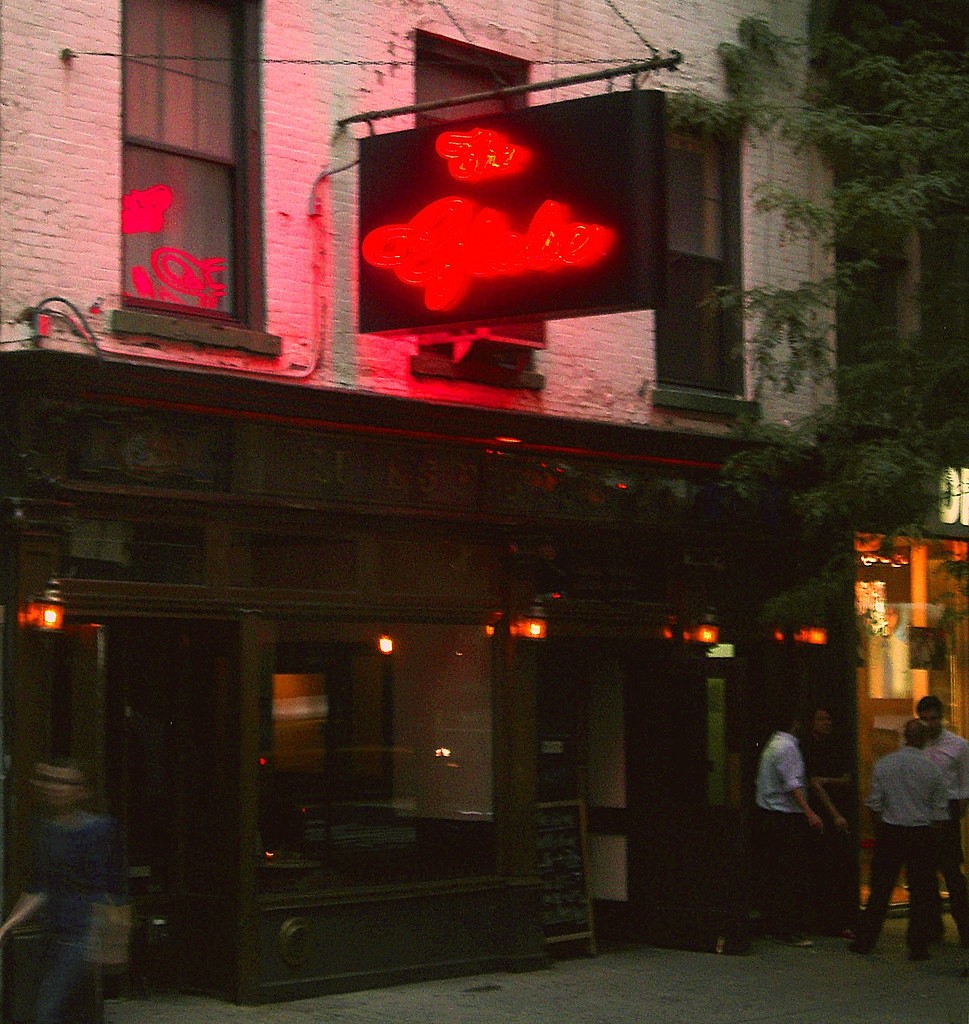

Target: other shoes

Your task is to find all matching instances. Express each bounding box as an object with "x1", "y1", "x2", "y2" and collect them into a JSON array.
[
  {"x1": 849, "y1": 942, "x2": 874, "y2": 954},
  {"x1": 780, "y1": 935, "x2": 813, "y2": 948},
  {"x1": 841, "y1": 927, "x2": 857, "y2": 940},
  {"x1": 907, "y1": 949, "x2": 929, "y2": 960}
]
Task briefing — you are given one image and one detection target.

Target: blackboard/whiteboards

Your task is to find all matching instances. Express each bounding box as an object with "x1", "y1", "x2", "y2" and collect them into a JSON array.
[{"x1": 535, "y1": 799, "x2": 595, "y2": 944}]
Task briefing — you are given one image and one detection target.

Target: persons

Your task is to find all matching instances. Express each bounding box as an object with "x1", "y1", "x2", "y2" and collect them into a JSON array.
[
  {"x1": 0, "y1": 750, "x2": 133, "y2": 1024},
  {"x1": 755, "y1": 696, "x2": 969, "y2": 961}
]
